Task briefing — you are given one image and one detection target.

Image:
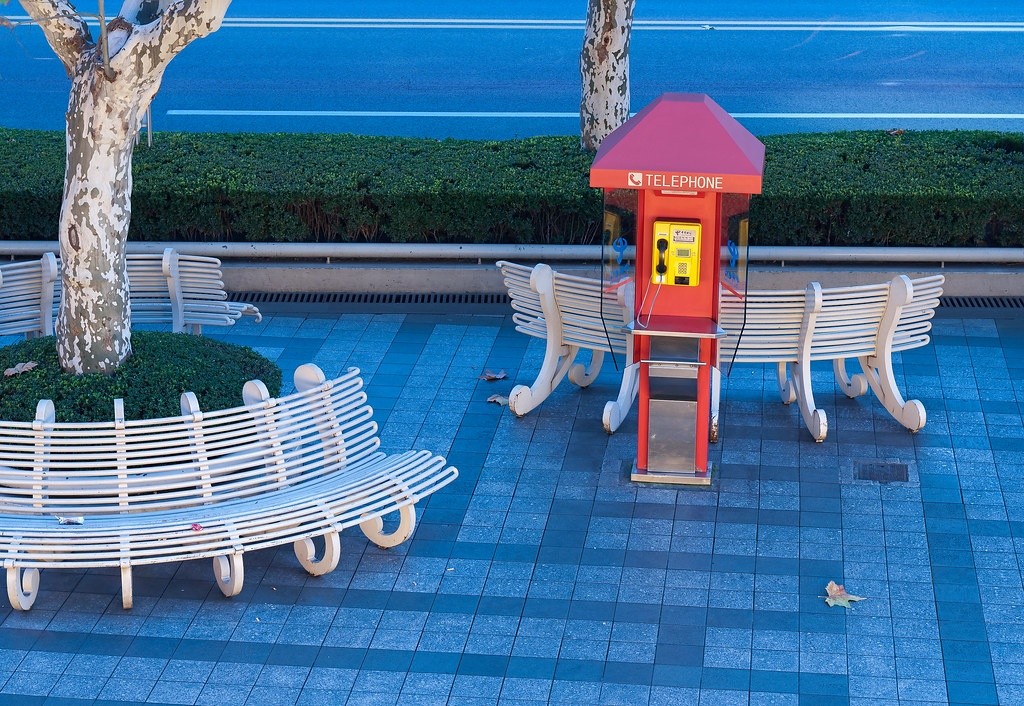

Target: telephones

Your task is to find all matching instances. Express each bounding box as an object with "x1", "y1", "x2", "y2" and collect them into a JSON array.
[{"x1": 655, "y1": 226, "x2": 696, "y2": 283}]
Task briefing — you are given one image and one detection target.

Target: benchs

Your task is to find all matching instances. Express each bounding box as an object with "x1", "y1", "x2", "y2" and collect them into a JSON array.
[
  {"x1": 0, "y1": 248, "x2": 461, "y2": 612},
  {"x1": 496, "y1": 259, "x2": 945, "y2": 443}
]
